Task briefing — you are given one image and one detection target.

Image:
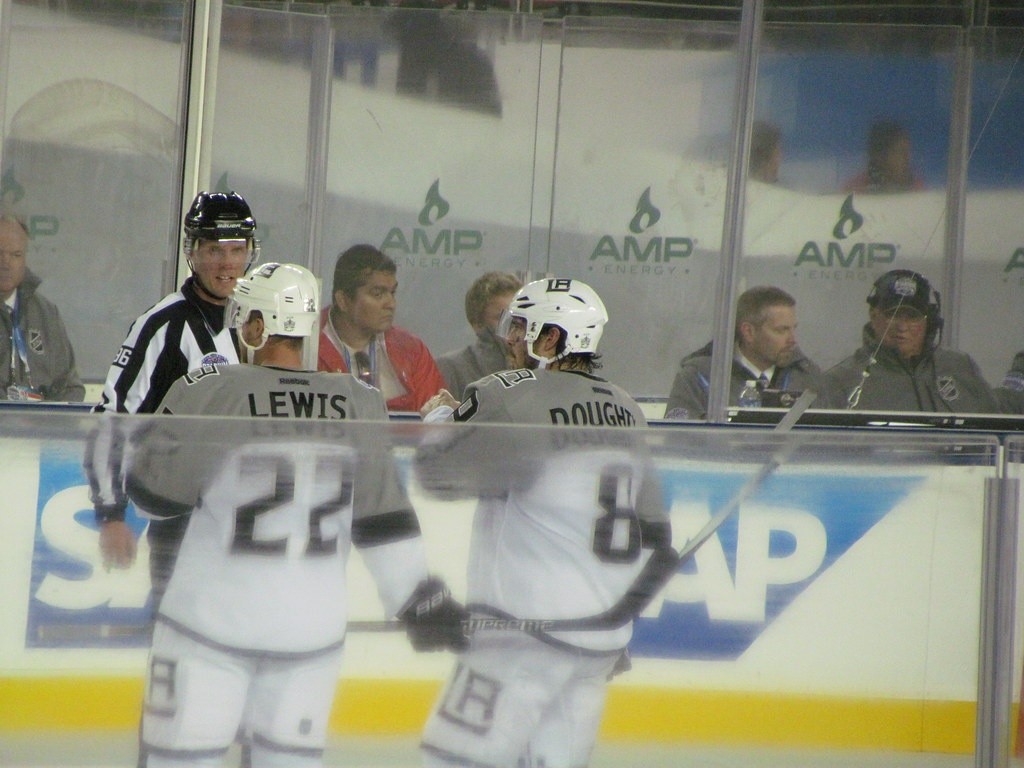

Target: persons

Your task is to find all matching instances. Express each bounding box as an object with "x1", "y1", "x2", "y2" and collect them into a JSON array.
[
  {"x1": 435, "y1": 272, "x2": 525, "y2": 402},
  {"x1": 331, "y1": 1, "x2": 503, "y2": 114},
  {"x1": 666, "y1": 286, "x2": 848, "y2": 427},
  {"x1": 822, "y1": 270, "x2": 1005, "y2": 452},
  {"x1": 123, "y1": 261, "x2": 468, "y2": 767},
  {"x1": 316, "y1": 245, "x2": 446, "y2": 411},
  {"x1": 843, "y1": 122, "x2": 922, "y2": 192},
  {"x1": 414, "y1": 278, "x2": 675, "y2": 768},
  {"x1": 0, "y1": 214, "x2": 87, "y2": 402},
  {"x1": 85, "y1": 191, "x2": 258, "y2": 606},
  {"x1": 749, "y1": 123, "x2": 782, "y2": 183}
]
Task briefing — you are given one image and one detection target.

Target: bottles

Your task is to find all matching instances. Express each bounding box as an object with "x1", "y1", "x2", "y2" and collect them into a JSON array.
[{"x1": 738, "y1": 379, "x2": 762, "y2": 407}]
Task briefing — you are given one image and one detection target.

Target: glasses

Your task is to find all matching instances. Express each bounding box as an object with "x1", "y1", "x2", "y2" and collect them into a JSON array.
[{"x1": 874, "y1": 310, "x2": 927, "y2": 325}]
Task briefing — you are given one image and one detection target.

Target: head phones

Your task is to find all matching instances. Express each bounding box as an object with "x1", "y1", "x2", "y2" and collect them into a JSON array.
[{"x1": 867, "y1": 268, "x2": 942, "y2": 345}]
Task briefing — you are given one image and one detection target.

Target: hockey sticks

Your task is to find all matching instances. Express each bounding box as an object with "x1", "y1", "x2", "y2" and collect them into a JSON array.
[
  {"x1": 685, "y1": 383, "x2": 823, "y2": 560},
  {"x1": 35, "y1": 544, "x2": 681, "y2": 642}
]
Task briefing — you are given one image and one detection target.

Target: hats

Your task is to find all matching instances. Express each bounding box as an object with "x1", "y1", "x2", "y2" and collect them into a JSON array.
[{"x1": 871, "y1": 269, "x2": 932, "y2": 316}]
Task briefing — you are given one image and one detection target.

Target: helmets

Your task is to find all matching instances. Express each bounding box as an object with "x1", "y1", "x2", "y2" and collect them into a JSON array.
[
  {"x1": 184, "y1": 192, "x2": 258, "y2": 239},
  {"x1": 508, "y1": 278, "x2": 608, "y2": 364},
  {"x1": 224, "y1": 262, "x2": 320, "y2": 350}
]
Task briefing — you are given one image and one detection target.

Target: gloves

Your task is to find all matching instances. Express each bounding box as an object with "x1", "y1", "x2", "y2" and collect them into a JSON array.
[{"x1": 396, "y1": 574, "x2": 470, "y2": 653}]
